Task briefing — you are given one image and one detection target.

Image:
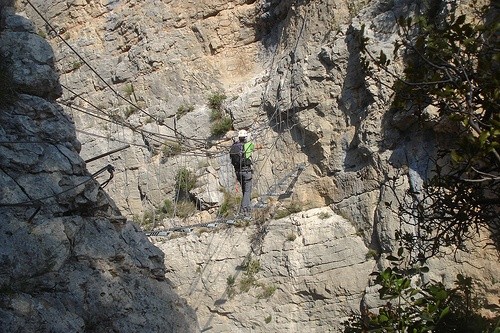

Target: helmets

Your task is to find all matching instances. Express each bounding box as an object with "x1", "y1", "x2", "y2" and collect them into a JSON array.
[{"x1": 237, "y1": 129, "x2": 248, "y2": 137}]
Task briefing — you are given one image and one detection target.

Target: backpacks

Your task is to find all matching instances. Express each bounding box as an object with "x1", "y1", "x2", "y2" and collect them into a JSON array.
[{"x1": 229, "y1": 142, "x2": 246, "y2": 168}]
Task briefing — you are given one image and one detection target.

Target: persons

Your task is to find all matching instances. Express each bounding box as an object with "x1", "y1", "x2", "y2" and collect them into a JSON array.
[{"x1": 235, "y1": 129, "x2": 263, "y2": 220}]
